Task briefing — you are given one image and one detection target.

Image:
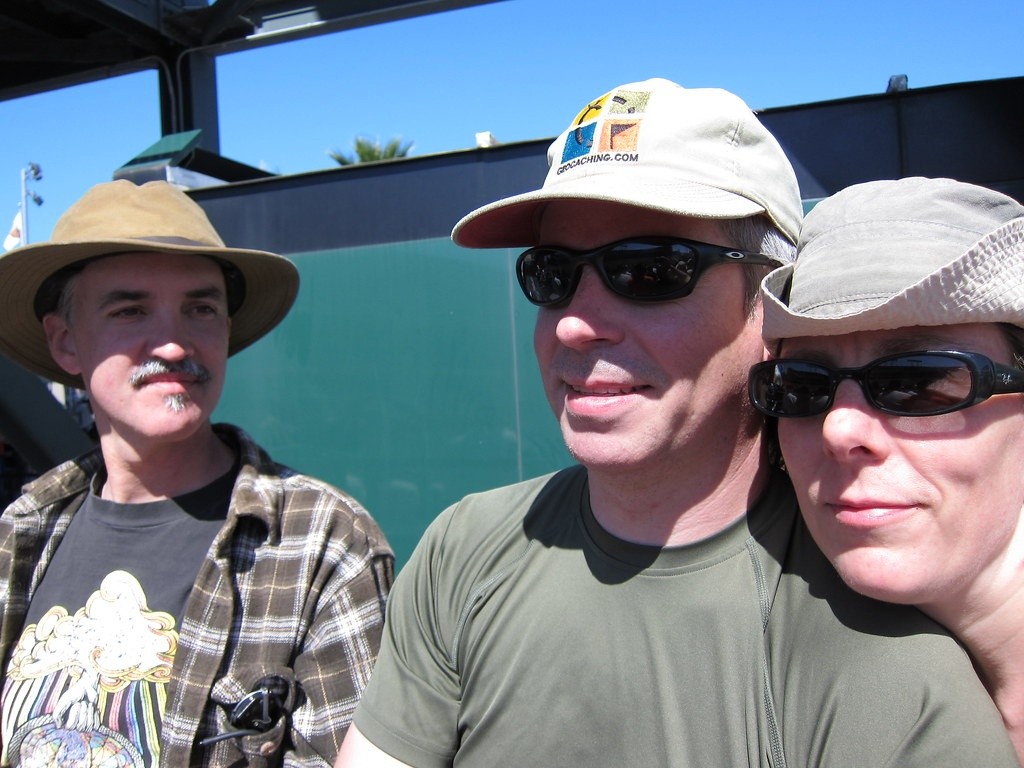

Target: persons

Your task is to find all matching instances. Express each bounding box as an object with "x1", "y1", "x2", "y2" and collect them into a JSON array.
[
  {"x1": 748, "y1": 179, "x2": 1024, "y2": 768},
  {"x1": 644, "y1": 255, "x2": 693, "y2": 296},
  {"x1": 0, "y1": 178, "x2": 395, "y2": 768},
  {"x1": 333, "y1": 76, "x2": 1018, "y2": 768}
]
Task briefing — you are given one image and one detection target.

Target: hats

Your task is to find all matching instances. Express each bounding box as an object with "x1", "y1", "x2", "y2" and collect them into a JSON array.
[
  {"x1": 450, "y1": 77, "x2": 803, "y2": 248},
  {"x1": 758, "y1": 176, "x2": 1024, "y2": 358},
  {"x1": 0, "y1": 177, "x2": 301, "y2": 391}
]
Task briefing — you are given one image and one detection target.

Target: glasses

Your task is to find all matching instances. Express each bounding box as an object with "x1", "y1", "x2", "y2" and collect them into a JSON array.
[
  {"x1": 515, "y1": 235, "x2": 782, "y2": 308},
  {"x1": 747, "y1": 351, "x2": 1024, "y2": 418}
]
